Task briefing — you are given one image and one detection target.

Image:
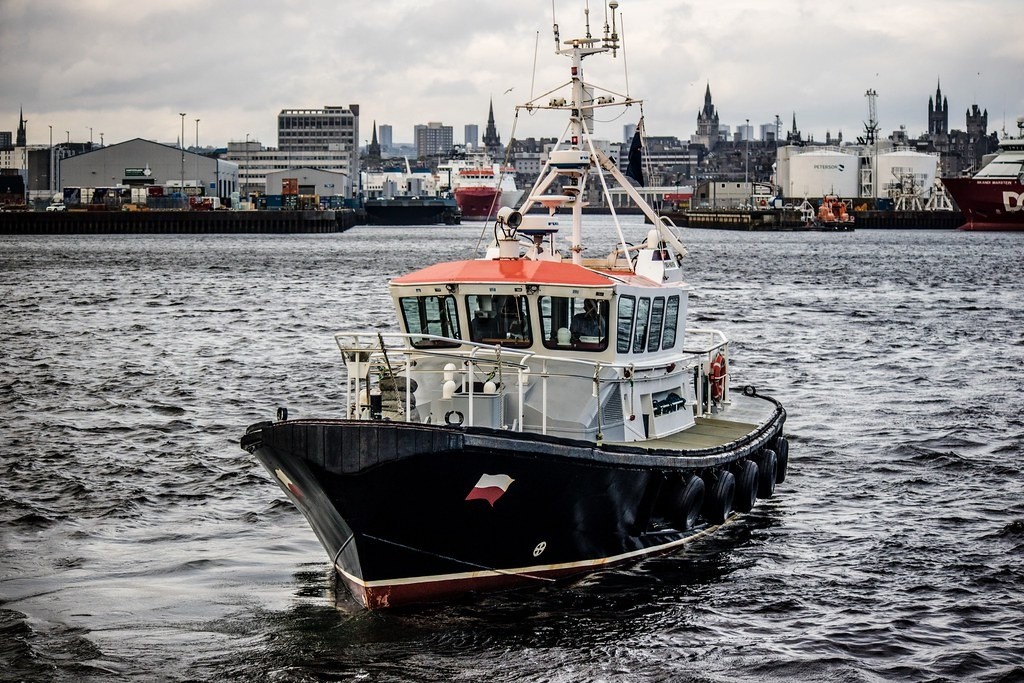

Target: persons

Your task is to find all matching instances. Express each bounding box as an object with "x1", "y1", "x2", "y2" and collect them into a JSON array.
[{"x1": 571, "y1": 299, "x2": 605, "y2": 337}]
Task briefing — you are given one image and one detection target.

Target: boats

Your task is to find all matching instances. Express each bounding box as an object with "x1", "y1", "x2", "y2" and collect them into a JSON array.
[
  {"x1": 790, "y1": 190, "x2": 820, "y2": 234},
  {"x1": 454, "y1": 156, "x2": 526, "y2": 222},
  {"x1": 236, "y1": 3, "x2": 789, "y2": 616},
  {"x1": 819, "y1": 185, "x2": 857, "y2": 232}
]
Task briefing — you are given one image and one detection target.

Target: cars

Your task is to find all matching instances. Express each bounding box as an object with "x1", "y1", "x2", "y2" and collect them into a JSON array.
[{"x1": 45, "y1": 203, "x2": 69, "y2": 213}]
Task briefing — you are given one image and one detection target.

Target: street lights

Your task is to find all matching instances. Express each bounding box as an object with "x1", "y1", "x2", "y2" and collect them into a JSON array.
[
  {"x1": 744, "y1": 119, "x2": 750, "y2": 181},
  {"x1": 22, "y1": 119, "x2": 105, "y2": 195},
  {"x1": 195, "y1": 118, "x2": 201, "y2": 180},
  {"x1": 243, "y1": 131, "x2": 251, "y2": 192},
  {"x1": 774, "y1": 112, "x2": 780, "y2": 146},
  {"x1": 179, "y1": 111, "x2": 188, "y2": 191}
]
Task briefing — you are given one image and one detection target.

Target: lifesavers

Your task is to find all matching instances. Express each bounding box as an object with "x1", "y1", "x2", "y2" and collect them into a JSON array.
[
  {"x1": 736, "y1": 459, "x2": 761, "y2": 514},
  {"x1": 757, "y1": 447, "x2": 777, "y2": 499},
  {"x1": 707, "y1": 471, "x2": 736, "y2": 525},
  {"x1": 775, "y1": 435, "x2": 789, "y2": 483},
  {"x1": 706, "y1": 351, "x2": 731, "y2": 405},
  {"x1": 680, "y1": 475, "x2": 706, "y2": 530}
]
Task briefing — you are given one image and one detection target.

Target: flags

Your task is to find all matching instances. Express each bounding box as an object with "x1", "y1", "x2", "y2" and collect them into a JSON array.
[{"x1": 626, "y1": 125, "x2": 644, "y2": 187}]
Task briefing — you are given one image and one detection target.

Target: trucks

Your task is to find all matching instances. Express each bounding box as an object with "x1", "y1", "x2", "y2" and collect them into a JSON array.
[{"x1": 148, "y1": 184, "x2": 206, "y2": 197}]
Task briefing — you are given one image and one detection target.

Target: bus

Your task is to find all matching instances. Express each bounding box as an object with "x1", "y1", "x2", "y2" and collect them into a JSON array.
[
  {"x1": 0, "y1": 202, "x2": 30, "y2": 213},
  {"x1": 62, "y1": 186, "x2": 131, "y2": 210}
]
